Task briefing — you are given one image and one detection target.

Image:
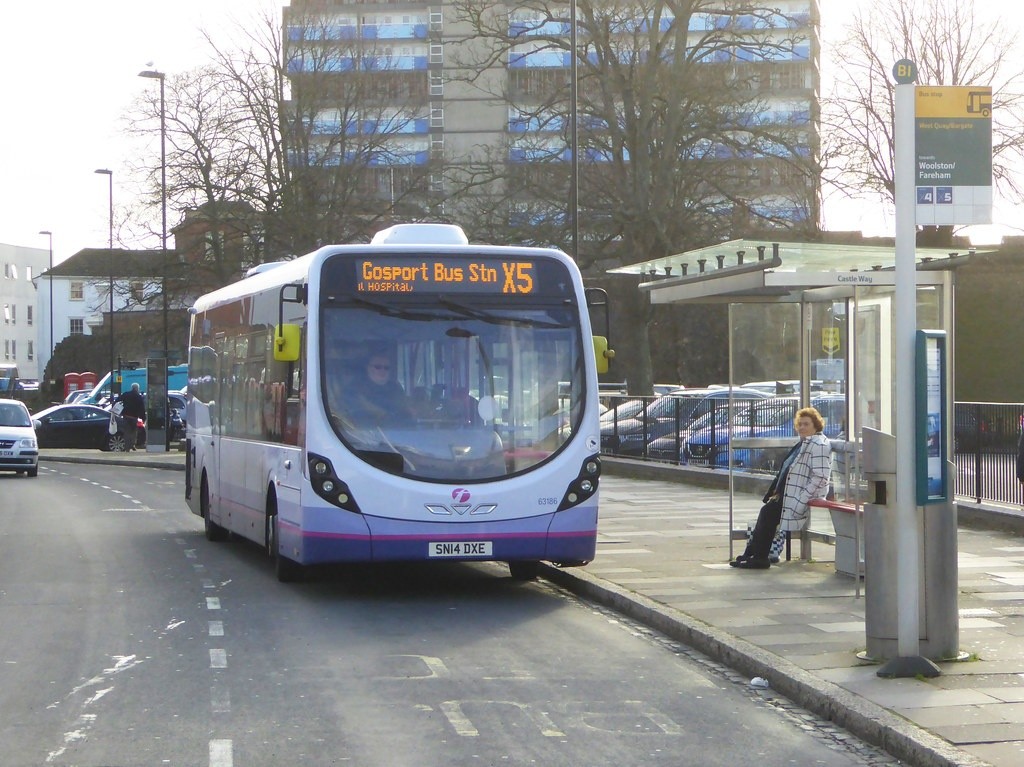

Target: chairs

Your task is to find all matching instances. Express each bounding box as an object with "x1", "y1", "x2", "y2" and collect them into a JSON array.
[{"x1": 333, "y1": 359, "x2": 367, "y2": 389}]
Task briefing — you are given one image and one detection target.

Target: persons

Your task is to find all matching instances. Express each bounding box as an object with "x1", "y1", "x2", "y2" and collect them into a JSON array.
[
  {"x1": 729, "y1": 405, "x2": 831, "y2": 574},
  {"x1": 170, "y1": 408, "x2": 185, "y2": 431},
  {"x1": 351, "y1": 344, "x2": 413, "y2": 428},
  {"x1": 112, "y1": 381, "x2": 146, "y2": 448}
]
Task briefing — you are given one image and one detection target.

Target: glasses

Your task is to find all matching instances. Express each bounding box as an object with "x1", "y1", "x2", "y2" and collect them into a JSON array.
[{"x1": 370, "y1": 363, "x2": 391, "y2": 370}]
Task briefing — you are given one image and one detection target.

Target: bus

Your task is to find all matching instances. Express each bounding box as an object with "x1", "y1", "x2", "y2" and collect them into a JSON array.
[{"x1": 185, "y1": 222, "x2": 617, "y2": 580}]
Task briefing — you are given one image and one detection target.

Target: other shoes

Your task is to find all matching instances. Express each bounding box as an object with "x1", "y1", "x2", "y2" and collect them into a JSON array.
[
  {"x1": 126, "y1": 444, "x2": 136, "y2": 452},
  {"x1": 729, "y1": 555, "x2": 770, "y2": 569}
]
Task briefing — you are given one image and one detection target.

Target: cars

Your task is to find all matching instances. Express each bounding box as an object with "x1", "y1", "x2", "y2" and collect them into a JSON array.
[
  {"x1": 168, "y1": 388, "x2": 186, "y2": 428},
  {"x1": 30, "y1": 402, "x2": 147, "y2": 452},
  {"x1": 486, "y1": 373, "x2": 849, "y2": 472}
]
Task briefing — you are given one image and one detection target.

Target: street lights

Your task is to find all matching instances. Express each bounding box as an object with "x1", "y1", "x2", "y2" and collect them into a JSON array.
[
  {"x1": 39, "y1": 231, "x2": 53, "y2": 380},
  {"x1": 137, "y1": 70, "x2": 169, "y2": 452},
  {"x1": 96, "y1": 167, "x2": 114, "y2": 408}
]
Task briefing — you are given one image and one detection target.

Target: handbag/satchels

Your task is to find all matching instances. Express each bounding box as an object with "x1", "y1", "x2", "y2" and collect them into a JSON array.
[
  {"x1": 109, "y1": 412, "x2": 118, "y2": 435},
  {"x1": 746, "y1": 518, "x2": 787, "y2": 560},
  {"x1": 109, "y1": 401, "x2": 125, "y2": 415}
]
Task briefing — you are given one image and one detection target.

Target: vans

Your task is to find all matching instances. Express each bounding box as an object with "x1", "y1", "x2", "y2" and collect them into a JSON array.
[{"x1": 0, "y1": 399, "x2": 42, "y2": 478}]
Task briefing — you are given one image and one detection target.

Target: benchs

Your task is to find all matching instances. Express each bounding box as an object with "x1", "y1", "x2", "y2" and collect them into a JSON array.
[{"x1": 808, "y1": 440, "x2": 864, "y2": 514}]
